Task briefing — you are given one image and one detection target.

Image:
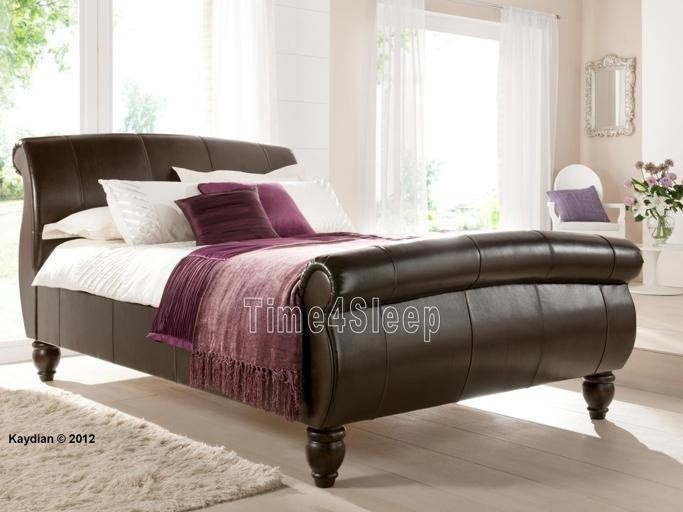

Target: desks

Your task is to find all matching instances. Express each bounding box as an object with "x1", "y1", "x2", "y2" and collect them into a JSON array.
[{"x1": 629, "y1": 238, "x2": 682, "y2": 299}]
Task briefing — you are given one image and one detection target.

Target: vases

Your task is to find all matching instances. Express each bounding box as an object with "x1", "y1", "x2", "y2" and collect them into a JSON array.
[{"x1": 646, "y1": 211, "x2": 673, "y2": 243}]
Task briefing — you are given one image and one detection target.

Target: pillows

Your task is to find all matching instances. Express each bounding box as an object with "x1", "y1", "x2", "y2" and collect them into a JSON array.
[
  {"x1": 544, "y1": 185, "x2": 611, "y2": 223},
  {"x1": 39, "y1": 155, "x2": 358, "y2": 253}
]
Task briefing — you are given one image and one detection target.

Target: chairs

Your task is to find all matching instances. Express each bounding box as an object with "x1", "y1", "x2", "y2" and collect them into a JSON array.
[{"x1": 543, "y1": 163, "x2": 627, "y2": 238}]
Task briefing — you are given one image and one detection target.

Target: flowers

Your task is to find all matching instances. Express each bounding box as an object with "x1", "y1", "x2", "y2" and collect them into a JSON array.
[{"x1": 624, "y1": 157, "x2": 682, "y2": 235}]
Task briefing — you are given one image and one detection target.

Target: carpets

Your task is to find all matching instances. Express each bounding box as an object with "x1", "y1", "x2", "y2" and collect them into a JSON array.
[{"x1": 0, "y1": 370, "x2": 289, "y2": 512}]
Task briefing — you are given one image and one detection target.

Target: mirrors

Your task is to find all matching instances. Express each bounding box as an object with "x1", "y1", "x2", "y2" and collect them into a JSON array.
[{"x1": 582, "y1": 52, "x2": 636, "y2": 138}]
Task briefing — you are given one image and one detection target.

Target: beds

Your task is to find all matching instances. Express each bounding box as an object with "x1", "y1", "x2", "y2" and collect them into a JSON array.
[{"x1": 8, "y1": 133, "x2": 648, "y2": 493}]
